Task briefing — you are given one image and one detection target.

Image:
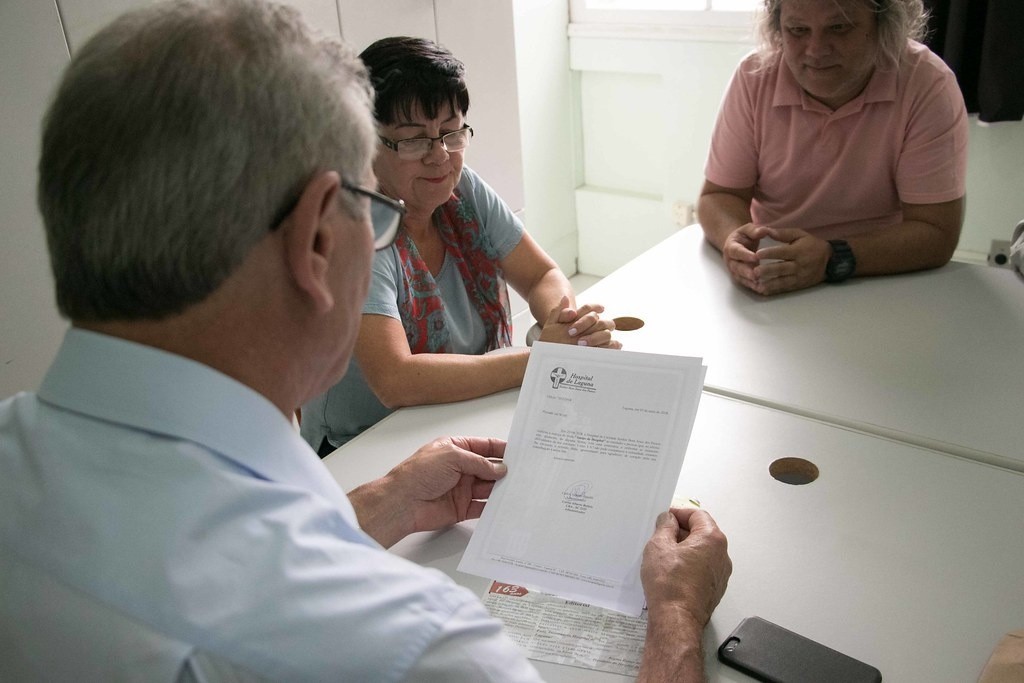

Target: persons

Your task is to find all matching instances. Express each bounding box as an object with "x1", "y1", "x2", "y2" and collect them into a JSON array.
[
  {"x1": 301, "y1": 36, "x2": 623, "y2": 461},
  {"x1": 698, "y1": 0, "x2": 971, "y2": 295},
  {"x1": 1, "y1": 0, "x2": 732, "y2": 682}
]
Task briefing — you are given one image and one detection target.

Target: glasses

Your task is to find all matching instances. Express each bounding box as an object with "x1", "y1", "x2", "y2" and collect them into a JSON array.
[
  {"x1": 268, "y1": 181, "x2": 409, "y2": 252},
  {"x1": 375, "y1": 123, "x2": 473, "y2": 161}
]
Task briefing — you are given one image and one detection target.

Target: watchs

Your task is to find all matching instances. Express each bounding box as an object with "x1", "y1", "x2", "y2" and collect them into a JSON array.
[{"x1": 826, "y1": 240, "x2": 856, "y2": 284}]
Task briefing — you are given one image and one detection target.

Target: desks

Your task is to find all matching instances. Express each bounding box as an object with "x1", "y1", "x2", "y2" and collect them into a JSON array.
[{"x1": 323, "y1": 223, "x2": 1024, "y2": 683}]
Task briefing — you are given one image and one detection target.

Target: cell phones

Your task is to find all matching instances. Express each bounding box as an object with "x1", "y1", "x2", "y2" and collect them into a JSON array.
[{"x1": 718, "y1": 616, "x2": 883, "y2": 683}]
